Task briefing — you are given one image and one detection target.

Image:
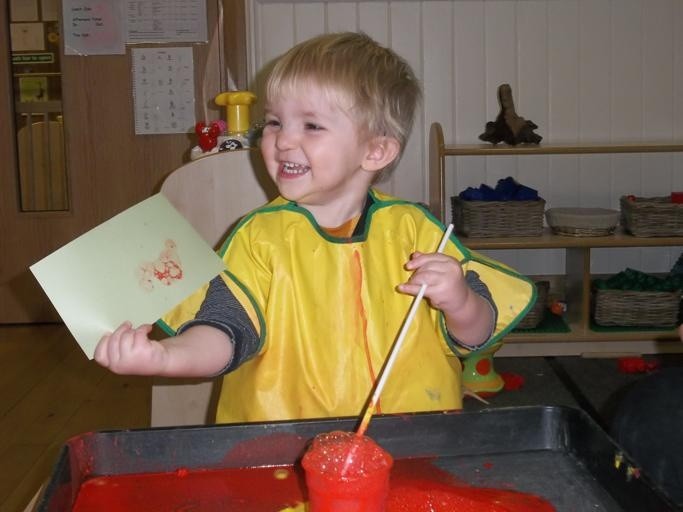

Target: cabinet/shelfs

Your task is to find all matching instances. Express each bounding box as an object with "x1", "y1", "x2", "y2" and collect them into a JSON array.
[{"x1": 428, "y1": 123, "x2": 683, "y2": 357}]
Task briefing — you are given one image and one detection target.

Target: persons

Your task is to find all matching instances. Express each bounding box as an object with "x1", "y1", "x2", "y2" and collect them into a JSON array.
[{"x1": 93, "y1": 30, "x2": 539, "y2": 429}]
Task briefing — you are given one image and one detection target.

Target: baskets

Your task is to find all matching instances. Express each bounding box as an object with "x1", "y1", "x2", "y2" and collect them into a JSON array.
[
  {"x1": 593, "y1": 286, "x2": 682, "y2": 329},
  {"x1": 451, "y1": 196, "x2": 683, "y2": 239},
  {"x1": 514, "y1": 280, "x2": 549, "y2": 329}
]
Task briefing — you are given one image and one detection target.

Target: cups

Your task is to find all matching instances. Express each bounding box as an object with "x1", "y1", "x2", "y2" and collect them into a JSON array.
[{"x1": 299, "y1": 442, "x2": 394, "y2": 511}]
